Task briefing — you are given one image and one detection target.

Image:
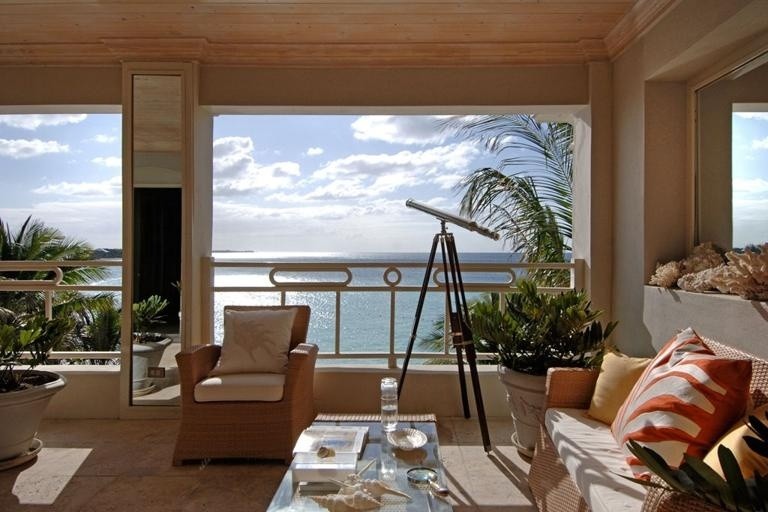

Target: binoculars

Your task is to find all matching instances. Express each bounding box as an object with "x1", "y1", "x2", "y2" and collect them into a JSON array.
[{"x1": 406, "y1": 197, "x2": 499, "y2": 241}]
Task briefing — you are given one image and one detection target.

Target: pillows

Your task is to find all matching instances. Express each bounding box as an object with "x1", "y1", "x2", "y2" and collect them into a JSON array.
[
  {"x1": 210, "y1": 308, "x2": 296, "y2": 375},
  {"x1": 703, "y1": 402, "x2": 768, "y2": 482},
  {"x1": 611, "y1": 326, "x2": 752, "y2": 487},
  {"x1": 587, "y1": 341, "x2": 657, "y2": 427}
]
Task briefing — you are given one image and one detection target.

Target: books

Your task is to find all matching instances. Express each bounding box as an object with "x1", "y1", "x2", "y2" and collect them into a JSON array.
[{"x1": 290, "y1": 422, "x2": 370, "y2": 492}]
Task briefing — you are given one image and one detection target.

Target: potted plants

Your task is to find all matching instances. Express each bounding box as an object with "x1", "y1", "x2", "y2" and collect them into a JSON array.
[
  {"x1": 422, "y1": 279, "x2": 620, "y2": 458},
  {"x1": 0, "y1": 312, "x2": 77, "y2": 470},
  {"x1": 132, "y1": 294, "x2": 172, "y2": 398}
]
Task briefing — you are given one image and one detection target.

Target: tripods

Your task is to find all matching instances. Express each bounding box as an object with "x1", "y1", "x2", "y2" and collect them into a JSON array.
[{"x1": 397, "y1": 230, "x2": 492, "y2": 455}]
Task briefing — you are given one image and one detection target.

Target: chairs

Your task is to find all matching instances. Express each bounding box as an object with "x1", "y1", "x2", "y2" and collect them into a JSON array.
[{"x1": 172, "y1": 305, "x2": 317, "y2": 467}]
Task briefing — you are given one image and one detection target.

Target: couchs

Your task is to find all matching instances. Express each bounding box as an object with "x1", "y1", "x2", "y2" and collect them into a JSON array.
[{"x1": 526, "y1": 326, "x2": 768, "y2": 512}]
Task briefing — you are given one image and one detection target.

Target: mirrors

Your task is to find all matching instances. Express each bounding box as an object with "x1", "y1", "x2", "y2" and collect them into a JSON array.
[{"x1": 130, "y1": 187, "x2": 185, "y2": 405}]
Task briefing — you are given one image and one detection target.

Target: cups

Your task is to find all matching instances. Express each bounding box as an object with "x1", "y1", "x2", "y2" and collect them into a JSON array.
[{"x1": 380, "y1": 376, "x2": 400, "y2": 435}]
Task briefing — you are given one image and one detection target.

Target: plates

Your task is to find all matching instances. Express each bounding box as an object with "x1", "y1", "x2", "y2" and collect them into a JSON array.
[{"x1": 387, "y1": 428, "x2": 428, "y2": 451}]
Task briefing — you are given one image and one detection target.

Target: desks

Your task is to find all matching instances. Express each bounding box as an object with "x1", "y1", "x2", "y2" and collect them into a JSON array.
[{"x1": 263, "y1": 413, "x2": 450, "y2": 512}]
